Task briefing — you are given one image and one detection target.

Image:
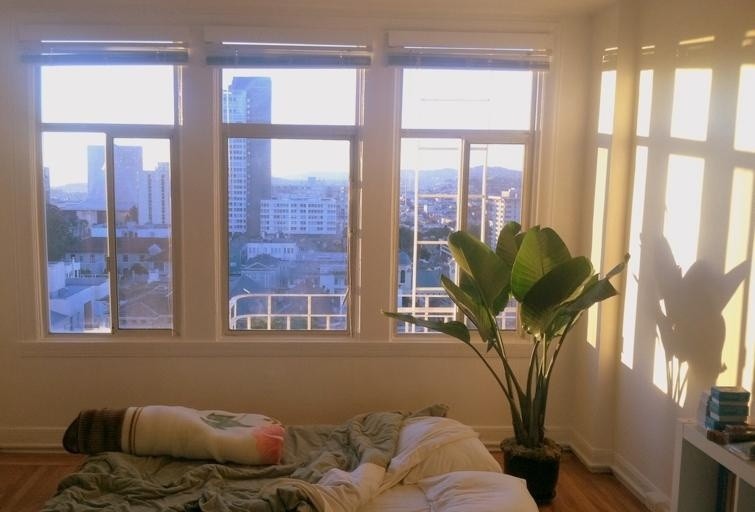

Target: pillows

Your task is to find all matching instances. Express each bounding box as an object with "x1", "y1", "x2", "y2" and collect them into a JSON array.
[{"x1": 394, "y1": 413, "x2": 539, "y2": 511}]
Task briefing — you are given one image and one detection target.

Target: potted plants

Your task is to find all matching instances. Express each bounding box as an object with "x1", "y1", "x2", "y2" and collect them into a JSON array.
[{"x1": 379, "y1": 220, "x2": 631, "y2": 507}]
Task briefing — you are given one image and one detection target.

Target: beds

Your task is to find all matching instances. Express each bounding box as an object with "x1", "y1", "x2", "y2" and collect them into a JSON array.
[{"x1": 41, "y1": 412, "x2": 542, "y2": 512}]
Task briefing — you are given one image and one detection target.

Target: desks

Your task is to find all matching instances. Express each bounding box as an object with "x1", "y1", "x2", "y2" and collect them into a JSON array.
[{"x1": 667, "y1": 417, "x2": 755, "y2": 511}]
